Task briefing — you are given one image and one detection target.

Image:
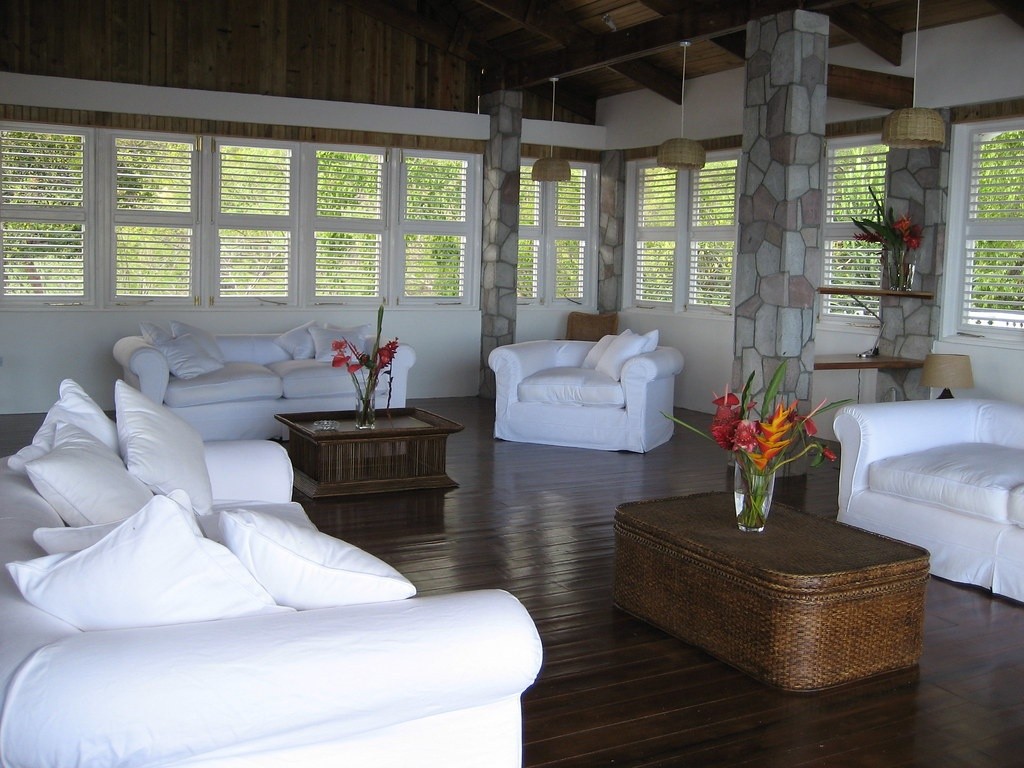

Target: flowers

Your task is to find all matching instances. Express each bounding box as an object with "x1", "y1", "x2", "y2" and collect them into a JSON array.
[
  {"x1": 658, "y1": 360, "x2": 856, "y2": 527},
  {"x1": 850, "y1": 185, "x2": 922, "y2": 290},
  {"x1": 331, "y1": 305, "x2": 402, "y2": 425}
]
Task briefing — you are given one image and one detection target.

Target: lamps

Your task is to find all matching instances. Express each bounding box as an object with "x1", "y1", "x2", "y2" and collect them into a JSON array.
[
  {"x1": 918, "y1": 353, "x2": 975, "y2": 399},
  {"x1": 532, "y1": 78, "x2": 571, "y2": 182},
  {"x1": 657, "y1": 41, "x2": 706, "y2": 168},
  {"x1": 881, "y1": 0, "x2": 946, "y2": 149}
]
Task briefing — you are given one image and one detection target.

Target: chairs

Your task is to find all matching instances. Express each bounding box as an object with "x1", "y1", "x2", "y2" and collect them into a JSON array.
[{"x1": 566, "y1": 311, "x2": 619, "y2": 343}]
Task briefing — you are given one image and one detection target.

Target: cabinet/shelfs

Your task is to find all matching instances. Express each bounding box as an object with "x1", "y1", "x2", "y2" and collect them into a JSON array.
[{"x1": 813, "y1": 286, "x2": 934, "y2": 369}]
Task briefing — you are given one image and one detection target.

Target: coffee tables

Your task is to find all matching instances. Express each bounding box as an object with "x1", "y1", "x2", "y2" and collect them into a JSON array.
[
  {"x1": 611, "y1": 493, "x2": 931, "y2": 694},
  {"x1": 274, "y1": 406, "x2": 466, "y2": 499}
]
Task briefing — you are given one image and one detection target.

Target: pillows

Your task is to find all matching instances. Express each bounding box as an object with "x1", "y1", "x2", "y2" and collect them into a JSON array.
[
  {"x1": 272, "y1": 320, "x2": 318, "y2": 360},
  {"x1": 0, "y1": 442, "x2": 543, "y2": 768},
  {"x1": 169, "y1": 319, "x2": 225, "y2": 363},
  {"x1": 154, "y1": 334, "x2": 224, "y2": 381},
  {"x1": 218, "y1": 508, "x2": 417, "y2": 612},
  {"x1": 308, "y1": 322, "x2": 372, "y2": 362},
  {"x1": 24, "y1": 420, "x2": 157, "y2": 527},
  {"x1": 4, "y1": 495, "x2": 297, "y2": 631},
  {"x1": 137, "y1": 321, "x2": 173, "y2": 345},
  {"x1": 33, "y1": 489, "x2": 194, "y2": 555},
  {"x1": 580, "y1": 328, "x2": 660, "y2": 382},
  {"x1": 5, "y1": 378, "x2": 120, "y2": 474},
  {"x1": 115, "y1": 380, "x2": 212, "y2": 516}
]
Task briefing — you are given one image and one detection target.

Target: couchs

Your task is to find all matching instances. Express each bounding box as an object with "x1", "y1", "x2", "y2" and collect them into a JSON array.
[
  {"x1": 488, "y1": 340, "x2": 684, "y2": 453},
  {"x1": 832, "y1": 398, "x2": 1024, "y2": 603},
  {"x1": 113, "y1": 333, "x2": 417, "y2": 442}
]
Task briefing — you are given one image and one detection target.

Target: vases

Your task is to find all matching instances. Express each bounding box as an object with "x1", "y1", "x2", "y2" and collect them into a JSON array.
[
  {"x1": 734, "y1": 449, "x2": 779, "y2": 532},
  {"x1": 884, "y1": 249, "x2": 917, "y2": 292},
  {"x1": 347, "y1": 362, "x2": 378, "y2": 429}
]
